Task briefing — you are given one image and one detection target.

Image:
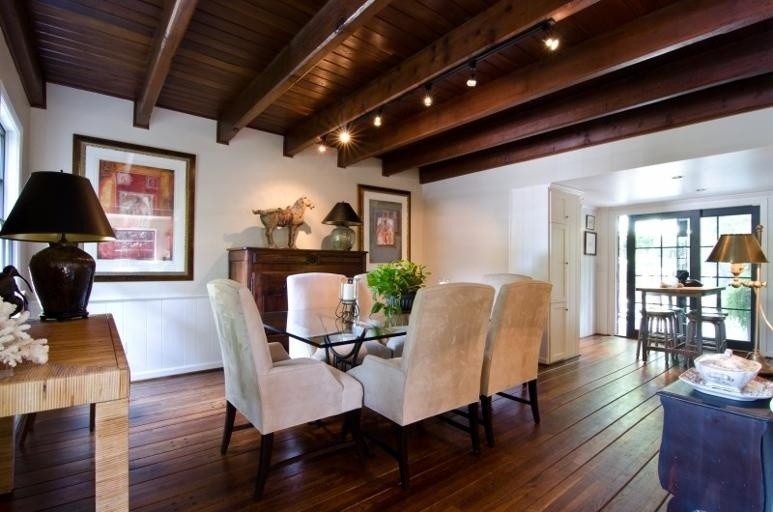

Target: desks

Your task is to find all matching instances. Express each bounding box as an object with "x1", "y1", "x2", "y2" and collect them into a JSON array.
[
  {"x1": 636, "y1": 286, "x2": 726, "y2": 369},
  {"x1": 260, "y1": 307, "x2": 408, "y2": 463}
]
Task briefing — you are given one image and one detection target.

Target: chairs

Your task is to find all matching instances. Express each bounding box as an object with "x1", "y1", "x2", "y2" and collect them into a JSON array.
[
  {"x1": 284, "y1": 270, "x2": 392, "y2": 370},
  {"x1": 205, "y1": 280, "x2": 365, "y2": 500},
  {"x1": 346, "y1": 282, "x2": 497, "y2": 491},
  {"x1": 352, "y1": 273, "x2": 395, "y2": 324},
  {"x1": 449, "y1": 280, "x2": 553, "y2": 448}
]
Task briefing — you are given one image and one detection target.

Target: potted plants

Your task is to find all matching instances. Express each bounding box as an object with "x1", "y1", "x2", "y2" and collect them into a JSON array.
[{"x1": 366, "y1": 258, "x2": 432, "y2": 319}]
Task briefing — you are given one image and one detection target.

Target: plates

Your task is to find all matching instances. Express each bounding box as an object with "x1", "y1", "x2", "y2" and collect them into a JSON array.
[{"x1": 678, "y1": 366, "x2": 773, "y2": 401}]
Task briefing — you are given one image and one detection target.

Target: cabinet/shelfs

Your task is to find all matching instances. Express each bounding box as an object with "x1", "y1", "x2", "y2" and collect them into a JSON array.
[
  {"x1": 1, "y1": 313, "x2": 132, "y2": 512},
  {"x1": 548, "y1": 228, "x2": 581, "y2": 363},
  {"x1": 655, "y1": 377, "x2": 773, "y2": 511},
  {"x1": 550, "y1": 191, "x2": 582, "y2": 225},
  {"x1": 226, "y1": 247, "x2": 369, "y2": 351}
]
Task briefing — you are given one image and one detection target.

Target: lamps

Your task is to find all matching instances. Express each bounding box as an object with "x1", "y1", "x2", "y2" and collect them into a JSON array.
[
  {"x1": 543, "y1": 39, "x2": 563, "y2": 51},
  {"x1": 372, "y1": 116, "x2": 383, "y2": 129},
  {"x1": 317, "y1": 143, "x2": 330, "y2": 154},
  {"x1": 705, "y1": 233, "x2": 773, "y2": 375},
  {"x1": 323, "y1": 202, "x2": 362, "y2": 251},
  {"x1": 467, "y1": 79, "x2": 477, "y2": 87},
  {"x1": 335, "y1": 276, "x2": 359, "y2": 324},
  {"x1": 339, "y1": 130, "x2": 353, "y2": 145},
  {"x1": 2, "y1": 170, "x2": 118, "y2": 323},
  {"x1": 424, "y1": 99, "x2": 435, "y2": 107}
]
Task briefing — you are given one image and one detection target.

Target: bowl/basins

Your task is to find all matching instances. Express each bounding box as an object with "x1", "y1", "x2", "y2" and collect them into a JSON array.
[{"x1": 693, "y1": 348, "x2": 763, "y2": 393}]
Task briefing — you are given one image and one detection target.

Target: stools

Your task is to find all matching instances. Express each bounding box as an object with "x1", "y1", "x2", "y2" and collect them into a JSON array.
[
  {"x1": 683, "y1": 313, "x2": 725, "y2": 370},
  {"x1": 699, "y1": 310, "x2": 729, "y2": 355},
  {"x1": 635, "y1": 308, "x2": 678, "y2": 369},
  {"x1": 652, "y1": 307, "x2": 683, "y2": 346}
]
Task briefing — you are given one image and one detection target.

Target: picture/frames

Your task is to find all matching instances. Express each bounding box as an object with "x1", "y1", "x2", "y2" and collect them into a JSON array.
[
  {"x1": 583, "y1": 231, "x2": 597, "y2": 255},
  {"x1": 357, "y1": 184, "x2": 412, "y2": 272},
  {"x1": 72, "y1": 134, "x2": 196, "y2": 280},
  {"x1": 586, "y1": 215, "x2": 597, "y2": 229}
]
